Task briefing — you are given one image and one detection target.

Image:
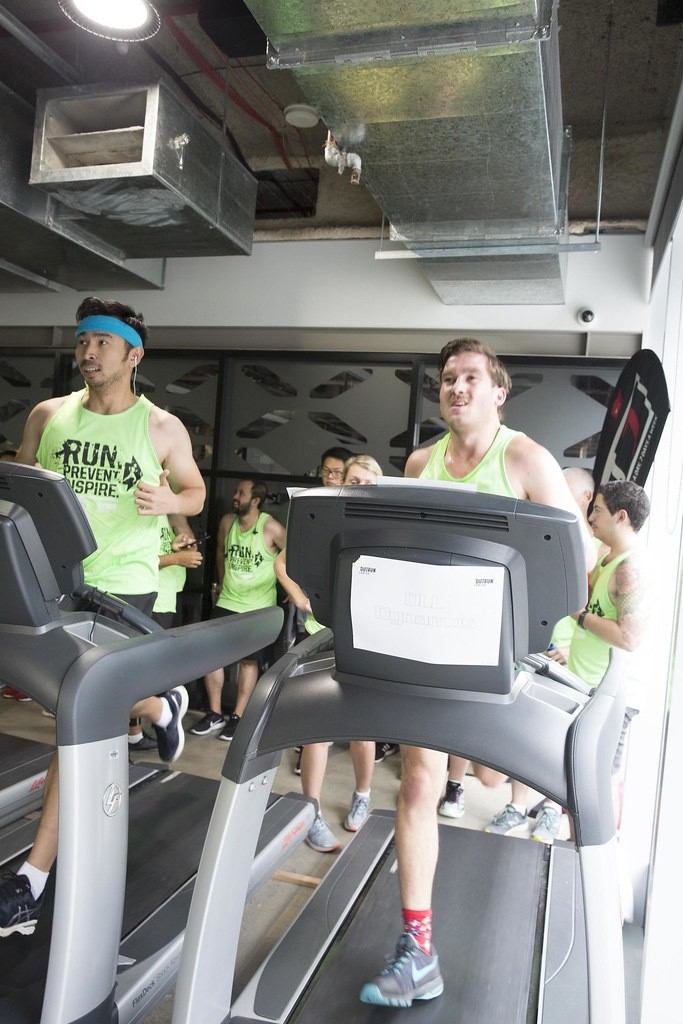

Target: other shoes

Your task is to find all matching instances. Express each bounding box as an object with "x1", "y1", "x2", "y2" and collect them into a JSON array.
[
  {"x1": 128, "y1": 731, "x2": 159, "y2": 751},
  {"x1": 295, "y1": 746, "x2": 304, "y2": 775},
  {"x1": 16, "y1": 690, "x2": 33, "y2": 701},
  {"x1": 1, "y1": 687, "x2": 19, "y2": 698},
  {"x1": 294, "y1": 745, "x2": 301, "y2": 752},
  {"x1": 0, "y1": 682, "x2": 6, "y2": 689},
  {"x1": 41, "y1": 707, "x2": 55, "y2": 717}
]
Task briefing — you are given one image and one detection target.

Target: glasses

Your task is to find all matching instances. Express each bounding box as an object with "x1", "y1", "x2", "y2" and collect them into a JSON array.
[{"x1": 320, "y1": 466, "x2": 344, "y2": 479}]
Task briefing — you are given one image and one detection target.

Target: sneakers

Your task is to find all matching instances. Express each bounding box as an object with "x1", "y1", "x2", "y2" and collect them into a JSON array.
[
  {"x1": 344, "y1": 791, "x2": 370, "y2": 832},
  {"x1": 151, "y1": 685, "x2": 190, "y2": 762},
  {"x1": 189, "y1": 710, "x2": 224, "y2": 735},
  {"x1": 530, "y1": 807, "x2": 561, "y2": 844},
  {"x1": 306, "y1": 813, "x2": 341, "y2": 852},
  {"x1": 0, "y1": 870, "x2": 46, "y2": 937},
  {"x1": 375, "y1": 742, "x2": 396, "y2": 762},
  {"x1": 438, "y1": 780, "x2": 464, "y2": 817},
  {"x1": 359, "y1": 932, "x2": 444, "y2": 1007},
  {"x1": 482, "y1": 804, "x2": 528, "y2": 834},
  {"x1": 218, "y1": 714, "x2": 241, "y2": 740}
]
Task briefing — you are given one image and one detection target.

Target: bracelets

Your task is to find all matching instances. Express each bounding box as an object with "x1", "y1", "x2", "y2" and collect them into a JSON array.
[{"x1": 577, "y1": 611, "x2": 588, "y2": 626}]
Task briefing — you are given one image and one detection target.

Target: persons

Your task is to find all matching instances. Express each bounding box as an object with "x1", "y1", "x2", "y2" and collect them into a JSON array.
[
  {"x1": 360, "y1": 338, "x2": 598, "y2": 1007},
  {"x1": 441, "y1": 467, "x2": 651, "y2": 843},
  {"x1": 274, "y1": 455, "x2": 383, "y2": 852},
  {"x1": 294, "y1": 446, "x2": 355, "y2": 773},
  {"x1": 0, "y1": 296, "x2": 206, "y2": 937},
  {"x1": 188, "y1": 480, "x2": 286, "y2": 741}
]
